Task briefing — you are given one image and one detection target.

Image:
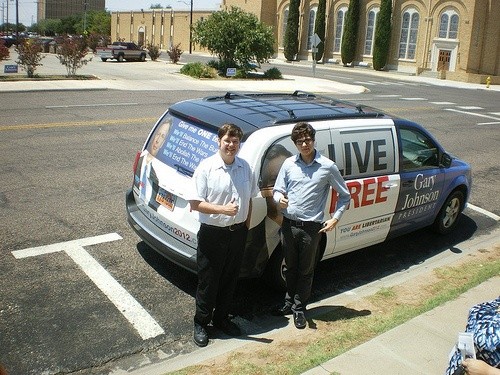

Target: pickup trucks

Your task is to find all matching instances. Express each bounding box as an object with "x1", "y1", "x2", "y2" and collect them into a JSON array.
[{"x1": 95, "y1": 41, "x2": 148, "y2": 63}]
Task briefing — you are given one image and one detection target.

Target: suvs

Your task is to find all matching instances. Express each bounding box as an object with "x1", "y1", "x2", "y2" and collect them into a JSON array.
[{"x1": 125, "y1": 90, "x2": 473, "y2": 296}]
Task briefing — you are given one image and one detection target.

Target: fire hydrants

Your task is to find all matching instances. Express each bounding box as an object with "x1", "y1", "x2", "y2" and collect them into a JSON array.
[{"x1": 486, "y1": 76, "x2": 491, "y2": 88}]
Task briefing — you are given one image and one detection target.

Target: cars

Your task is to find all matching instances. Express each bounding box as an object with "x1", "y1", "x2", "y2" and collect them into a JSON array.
[{"x1": 0, "y1": 30, "x2": 39, "y2": 40}]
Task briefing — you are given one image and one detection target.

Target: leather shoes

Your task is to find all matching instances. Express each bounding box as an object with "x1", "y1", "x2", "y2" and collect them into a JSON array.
[
  {"x1": 273, "y1": 303, "x2": 293, "y2": 316},
  {"x1": 214, "y1": 318, "x2": 241, "y2": 336},
  {"x1": 194, "y1": 322, "x2": 208, "y2": 346},
  {"x1": 294, "y1": 313, "x2": 306, "y2": 327}
]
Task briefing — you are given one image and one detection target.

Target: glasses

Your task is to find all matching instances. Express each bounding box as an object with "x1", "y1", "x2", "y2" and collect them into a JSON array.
[
  {"x1": 220, "y1": 140, "x2": 239, "y2": 146},
  {"x1": 296, "y1": 138, "x2": 313, "y2": 145}
]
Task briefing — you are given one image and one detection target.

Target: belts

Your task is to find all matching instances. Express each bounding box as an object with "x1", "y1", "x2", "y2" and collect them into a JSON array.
[
  {"x1": 201, "y1": 222, "x2": 246, "y2": 231},
  {"x1": 283, "y1": 217, "x2": 315, "y2": 226}
]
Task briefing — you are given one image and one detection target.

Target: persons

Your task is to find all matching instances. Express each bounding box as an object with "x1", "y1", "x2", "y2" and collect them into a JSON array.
[
  {"x1": 133, "y1": 118, "x2": 173, "y2": 211},
  {"x1": 243, "y1": 144, "x2": 292, "y2": 274},
  {"x1": 188, "y1": 122, "x2": 259, "y2": 347},
  {"x1": 272, "y1": 123, "x2": 350, "y2": 329},
  {"x1": 444, "y1": 296, "x2": 500, "y2": 375}
]
